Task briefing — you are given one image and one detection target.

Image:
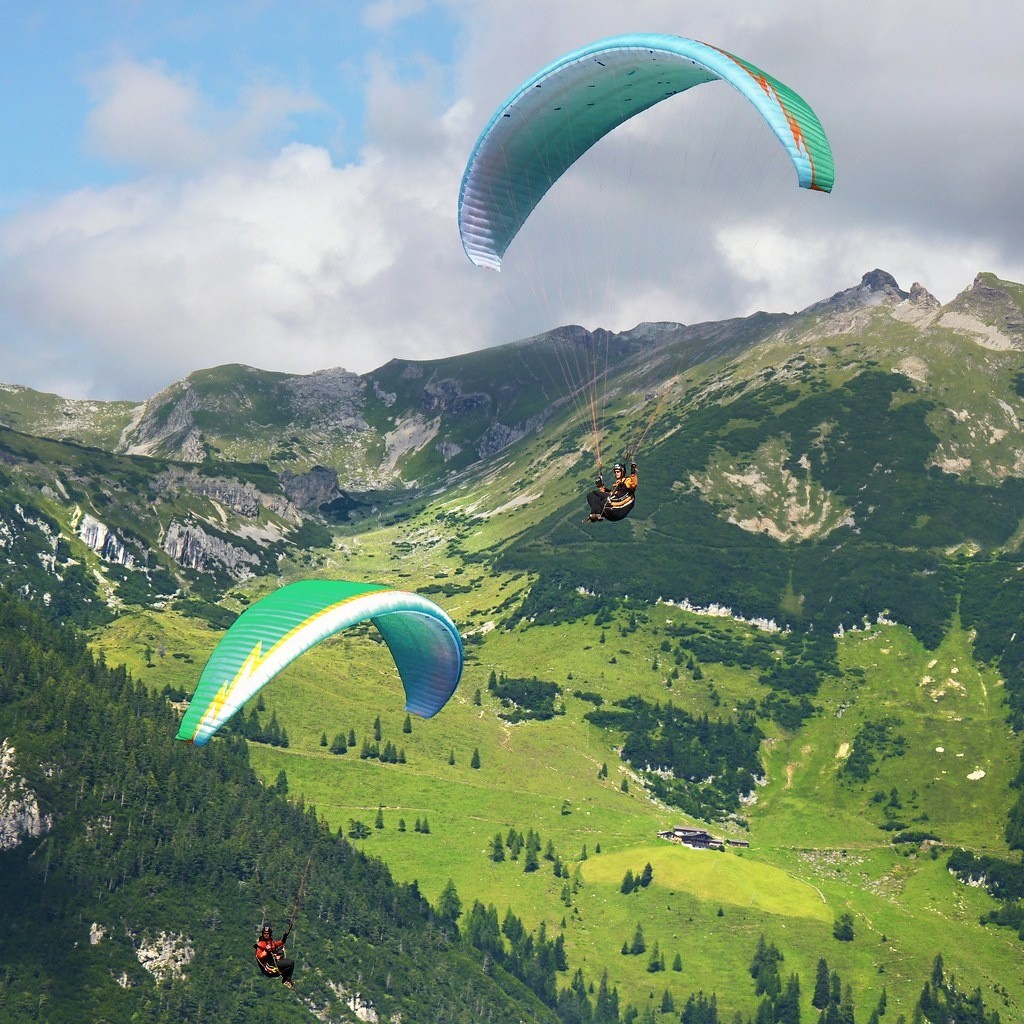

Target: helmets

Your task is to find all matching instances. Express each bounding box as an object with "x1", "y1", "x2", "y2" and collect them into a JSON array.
[
  {"x1": 262, "y1": 927, "x2": 272, "y2": 937},
  {"x1": 613, "y1": 462, "x2": 626, "y2": 478}
]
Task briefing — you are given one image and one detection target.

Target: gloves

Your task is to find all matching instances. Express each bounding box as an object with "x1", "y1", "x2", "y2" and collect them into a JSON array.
[
  {"x1": 630, "y1": 461, "x2": 637, "y2": 470},
  {"x1": 595, "y1": 477, "x2": 603, "y2": 487},
  {"x1": 265, "y1": 945, "x2": 272, "y2": 952},
  {"x1": 281, "y1": 937, "x2": 287, "y2": 942}
]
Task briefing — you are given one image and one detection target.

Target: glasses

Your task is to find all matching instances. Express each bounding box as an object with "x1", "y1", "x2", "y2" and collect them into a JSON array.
[
  {"x1": 263, "y1": 933, "x2": 270, "y2": 935},
  {"x1": 614, "y1": 470, "x2": 620, "y2": 473}
]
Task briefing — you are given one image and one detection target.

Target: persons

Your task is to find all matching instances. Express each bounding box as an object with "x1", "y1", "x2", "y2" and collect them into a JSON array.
[
  {"x1": 582, "y1": 460, "x2": 638, "y2": 526},
  {"x1": 255, "y1": 926, "x2": 295, "y2": 991}
]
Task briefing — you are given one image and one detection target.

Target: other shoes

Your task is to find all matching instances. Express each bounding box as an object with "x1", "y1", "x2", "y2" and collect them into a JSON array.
[
  {"x1": 583, "y1": 512, "x2": 603, "y2": 524},
  {"x1": 283, "y1": 981, "x2": 293, "y2": 989}
]
